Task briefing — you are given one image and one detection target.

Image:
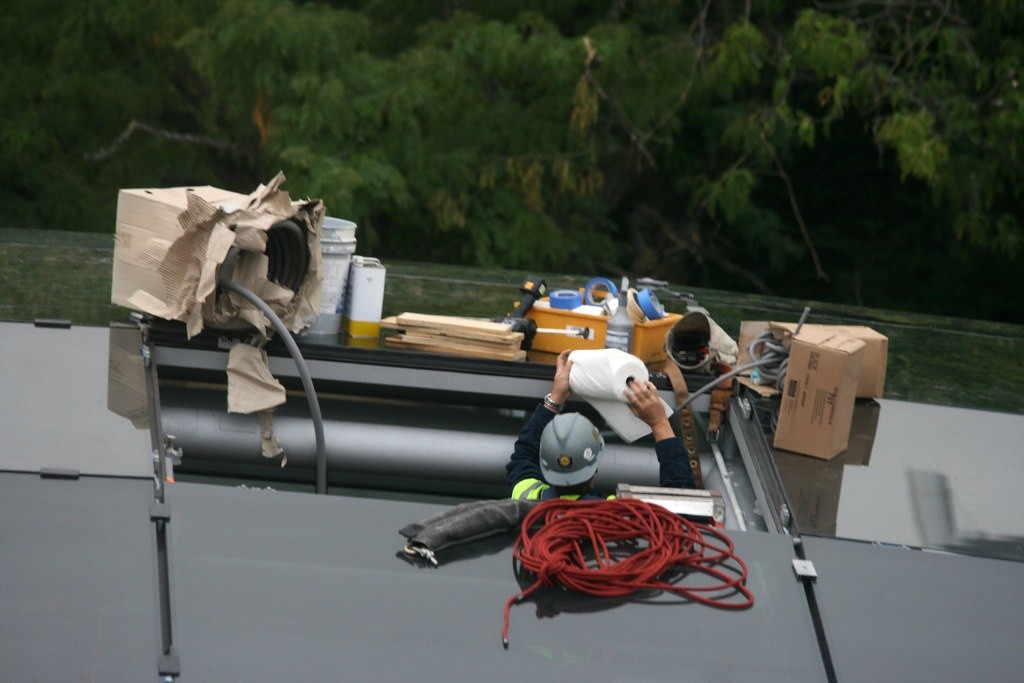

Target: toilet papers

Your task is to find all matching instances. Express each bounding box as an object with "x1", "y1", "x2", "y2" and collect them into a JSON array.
[{"x1": 568, "y1": 349, "x2": 674, "y2": 444}]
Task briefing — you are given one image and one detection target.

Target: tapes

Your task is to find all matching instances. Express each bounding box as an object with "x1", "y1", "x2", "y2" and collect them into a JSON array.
[
  {"x1": 638, "y1": 287, "x2": 663, "y2": 319},
  {"x1": 549, "y1": 289, "x2": 582, "y2": 310},
  {"x1": 586, "y1": 278, "x2": 617, "y2": 308}
]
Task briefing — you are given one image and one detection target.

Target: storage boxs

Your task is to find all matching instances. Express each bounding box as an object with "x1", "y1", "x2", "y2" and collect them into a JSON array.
[
  {"x1": 111, "y1": 172, "x2": 327, "y2": 333},
  {"x1": 772, "y1": 326, "x2": 865, "y2": 460},
  {"x1": 733, "y1": 320, "x2": 888, "y2": 396},
  {"x1": 515, "y1": 287, "x2": 683, "y2": 364}
]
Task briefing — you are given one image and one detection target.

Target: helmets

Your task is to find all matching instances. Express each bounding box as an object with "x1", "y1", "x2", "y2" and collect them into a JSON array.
[{"x1": 539, "y1": 412, "x2": 604, "y2": 486}]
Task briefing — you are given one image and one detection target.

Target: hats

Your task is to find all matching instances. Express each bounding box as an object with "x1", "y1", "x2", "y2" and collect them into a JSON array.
[{"x1": 666, "y1": 312, "x2": 711, "y2": 370}]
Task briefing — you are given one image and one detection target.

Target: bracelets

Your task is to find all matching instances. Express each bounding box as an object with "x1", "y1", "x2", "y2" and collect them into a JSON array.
[{"x1": 544, "y1": 393, "x2": 562, "y2": 412}]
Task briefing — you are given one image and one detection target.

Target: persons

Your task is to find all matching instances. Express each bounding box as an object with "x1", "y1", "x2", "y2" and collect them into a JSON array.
[{"x1": 507, "y1": 349, "x2": 696, "y2": 503}]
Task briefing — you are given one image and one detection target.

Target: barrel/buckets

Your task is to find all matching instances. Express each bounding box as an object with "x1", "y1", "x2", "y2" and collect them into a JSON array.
[
  {"x1": 310, "y1": 216, "x2": 357, "y2": 334},
  {"x1": 342, "y1": 256, "x2": 386, "y2": 340}
]
